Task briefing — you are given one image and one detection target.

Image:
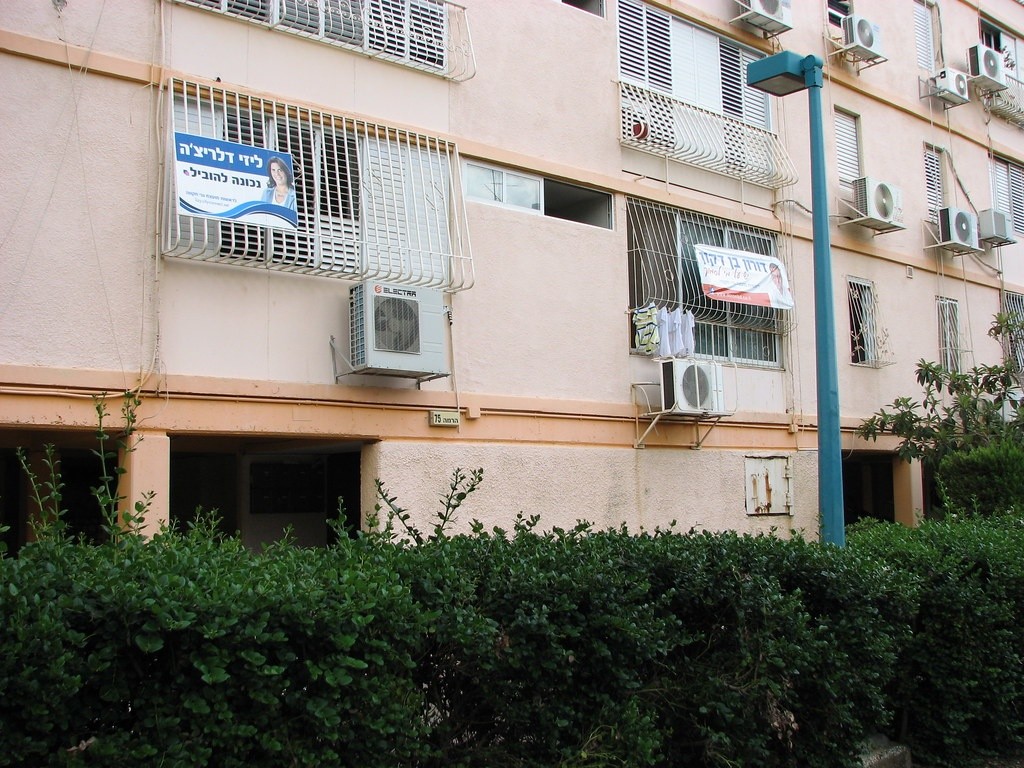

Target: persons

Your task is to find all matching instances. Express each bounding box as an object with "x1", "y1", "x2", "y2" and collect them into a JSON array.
[
  {"x1": 262, "y1": 156, "x2": 296, "y2": 212},
  {"x1": 768, "y1": 263, "x2": 794, "y2": 310}
]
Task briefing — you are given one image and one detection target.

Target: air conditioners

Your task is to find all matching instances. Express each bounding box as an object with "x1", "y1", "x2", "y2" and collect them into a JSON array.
[
  {"x1": 977, "y1": 209, "x2": 1012, "y2": 242},
  {"x1": 740, "y1": 0, "x2": 792, "y2": 31},
  {"x1": 344, "y1": 280, "x2": 446, "y2": 377},
  {"x1": 936, "y1": 68, "x2": 968, "y2": 104},
  {"x1": 965, "y1": 43, "x2": 1006, "y2": 90},
  {"x1": 659, "y1": 359, "x2": 724, "y2": 413},
  {"x1": 852, "y1": 176, "x2": 904, "y2": 230},
  {"x1": 938, "y1": 207, "x2": 979, "y2": 252},
  {"x1": 840, "y1": 13, "x2": 882, "y2": 59}
]
